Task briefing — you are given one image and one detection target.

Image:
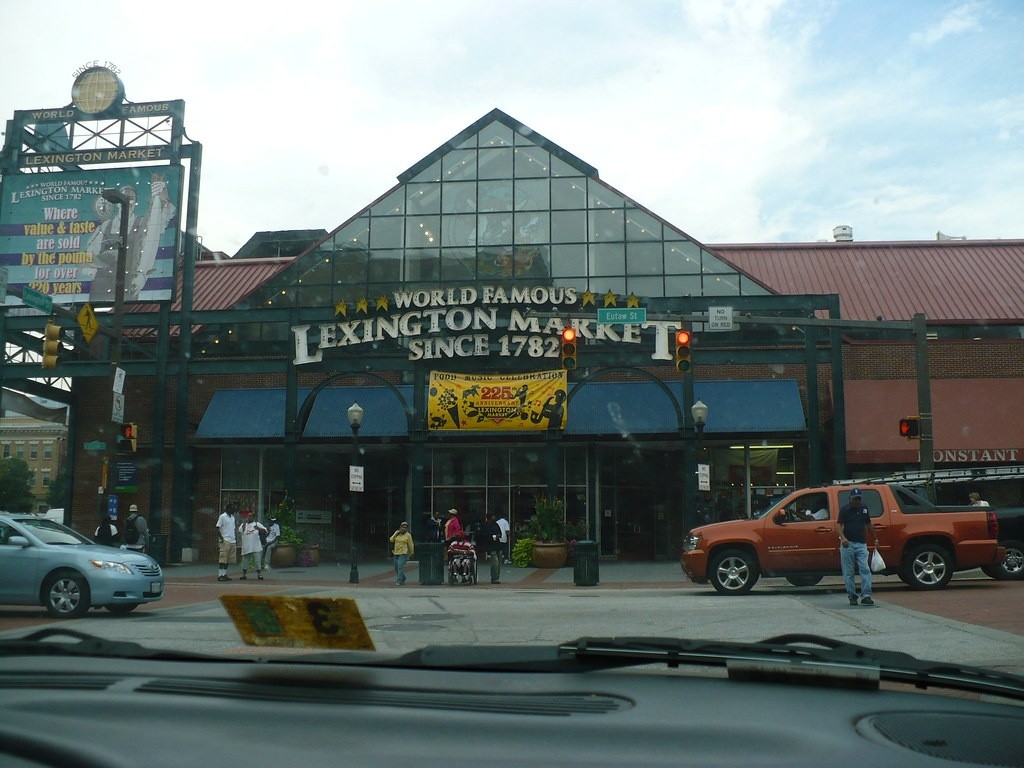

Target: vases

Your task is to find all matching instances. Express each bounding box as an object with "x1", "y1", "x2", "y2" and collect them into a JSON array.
[
  {"x1": 271, "y1": 545, "x2": 296, "y2": 568},
  {"x1": 533, "y1": 540, "x2": 567, "y2": 568},
  {"x1": 299, "y1": 545, "x2": 321, "y2": 566}
]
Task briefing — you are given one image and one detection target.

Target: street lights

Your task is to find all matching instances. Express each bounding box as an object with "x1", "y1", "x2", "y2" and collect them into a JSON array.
[
  {"x1": 346, "y1": 402, "x2": 361, "y2": 584},
  {"x1": 99, "y1": 189, "x2": 131, "y2": 545},
  {"x1": 690, "y1": 395, "x2": 708, "y2": 523}
]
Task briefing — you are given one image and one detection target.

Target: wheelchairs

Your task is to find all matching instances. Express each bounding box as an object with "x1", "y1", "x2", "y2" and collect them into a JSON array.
[{"x1": 447, "y1": 559, "x2": 479, "y2": 586}]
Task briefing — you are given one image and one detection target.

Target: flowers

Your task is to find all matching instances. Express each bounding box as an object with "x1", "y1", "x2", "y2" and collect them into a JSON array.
[
  {"x1": 511, "y1": 494, "x2": 590, "y2": 568},
  {"x1": 299, "y1": 543, "x2": 312, "y2": 568},
  {"x1": 241, "y1": 490, "x2": 303, "y2": 570}
]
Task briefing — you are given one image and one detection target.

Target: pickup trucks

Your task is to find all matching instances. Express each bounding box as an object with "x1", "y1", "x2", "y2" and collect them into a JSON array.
[
  {"x1": 681, "y1": 483, "x2": 1005, "y2": 596},
  {"x1": 981, "y1": 505, "x2": 1024, "y2": 580}
]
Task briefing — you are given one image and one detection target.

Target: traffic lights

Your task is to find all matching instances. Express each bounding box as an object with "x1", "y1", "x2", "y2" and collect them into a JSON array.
[
  {"x1": 899, "y1": 418, "x2": 919, "y2": 436},
  {"x1": 42, "y1": 322, "x2": 66, "y2": 370},
  {"x1": 562, "y1": 329, "x2": 578, "y2": 370},
  {"x1": 118, "y1": 422, "x2": 138, "y2": 454},
  {"x1": 675, "y1": 331, "x2": 693, "y2": 374}
]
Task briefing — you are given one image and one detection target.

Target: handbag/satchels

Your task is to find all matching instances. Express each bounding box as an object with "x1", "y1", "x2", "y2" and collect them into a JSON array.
[{"x1": 871, "y1": 547, "x2": 886, "y2": 572}]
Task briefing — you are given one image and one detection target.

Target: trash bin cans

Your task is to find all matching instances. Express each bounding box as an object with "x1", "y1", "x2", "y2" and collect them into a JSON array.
[
  {"x1": 417, "y1": 543, "x2": 445, "y2": 586},
  {"x1": 147, "y1": 533, "x2": 170, "y2": 568},
  {"x1": 574, "y1": 539, "x2": 600, "y2": 587}
]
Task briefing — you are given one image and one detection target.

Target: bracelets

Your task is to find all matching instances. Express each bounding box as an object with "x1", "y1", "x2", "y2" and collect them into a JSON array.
[{"x1": 873, "y1": 539, "x2": 878, "y2": 542}]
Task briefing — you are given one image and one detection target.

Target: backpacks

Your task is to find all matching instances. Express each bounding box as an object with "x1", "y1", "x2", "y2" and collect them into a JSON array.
[{"x1": 126, "y1": 515, "x2": 140, "y2": 544}]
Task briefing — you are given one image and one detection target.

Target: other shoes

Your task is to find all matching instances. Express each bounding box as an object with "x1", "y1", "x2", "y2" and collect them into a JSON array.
[
  {"x1": 239, "y1": 575, "x2": 248, "y2": 579},
  {"x1": 257, "y1": 574, "x2": 263, "y2": 580},
  {"x1": 861, "y1": 597, "x2": 873, "y2": 605},
  {"x1": 218, "y1": 575, "x2": 232, "y2": 582},
  {"x1": 396, "y1": 582, "x2": 402, "y2": 586},
  {"x1": 850, "y1": 597, "x2": 859, "y2": 606}
]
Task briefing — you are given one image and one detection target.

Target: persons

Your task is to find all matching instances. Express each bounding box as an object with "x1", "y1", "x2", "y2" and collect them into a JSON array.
[
  {"x1": 448, "y1": 531, "x2": 476, "y2": 582},
  {"x1": 789, "y1": 496, "x2": 829, "y2": 520},
  {"x1": 123, "y1": 505, "x2": 149, "y2": 552},
  {"x1": 427, "y1": 511, "x2": 443, "y2": 542},
  {"x1": 480, "y1": 512, "x2": 502, "y2": 584},
  {"x1": 495, "y1": 513, "x2": 512, "y2": 564},
  {"x1": 260, "y1": 516, "x2": 280, "y2": 569},
  {"x1": 443, "y1": 509, "x2": 461, "y2": 569},
  {"x1": 390, "y1": 522, "x2": 414, "y2": 585},
  {"x1": 836, "y1": 488, "x2": 879, "y2": 605},
  {"x1": 216, "y1": 504, "x2": 237, "y2": 581},
  {"x1": 94, "y1": 516, "x2": 118, "y2": 546},
  {"x1": 239, "y1": 513, "x2": 268, "y2": 579},
  {"x1": 969, "y1": 492, "x2": 989, "y2": 507}
]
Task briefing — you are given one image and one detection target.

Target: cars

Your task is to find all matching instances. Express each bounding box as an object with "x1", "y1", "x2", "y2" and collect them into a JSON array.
[{"x1": 0, "y1": 510, "x2": 166, "y2": 619}]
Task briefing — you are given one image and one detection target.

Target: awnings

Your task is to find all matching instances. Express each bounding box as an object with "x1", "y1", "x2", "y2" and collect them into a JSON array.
[
  {"x1": 828, "y1": 377, "x2": 1024, "y2": 463},
  {"x1": 195, "y1": 381, "x2": 808, "y2": 439}
]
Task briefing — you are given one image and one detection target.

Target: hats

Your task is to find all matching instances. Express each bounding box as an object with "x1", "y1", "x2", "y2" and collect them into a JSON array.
[
  {"x1": 129, "y1": 504, "x2": 138, "y2": 512},
  {"x1": 447, "y1": 509, "x2": 458, "y2": 515},
  {"x1": 848, "y1": 487, "x2": 863, "y2": 499}
]
